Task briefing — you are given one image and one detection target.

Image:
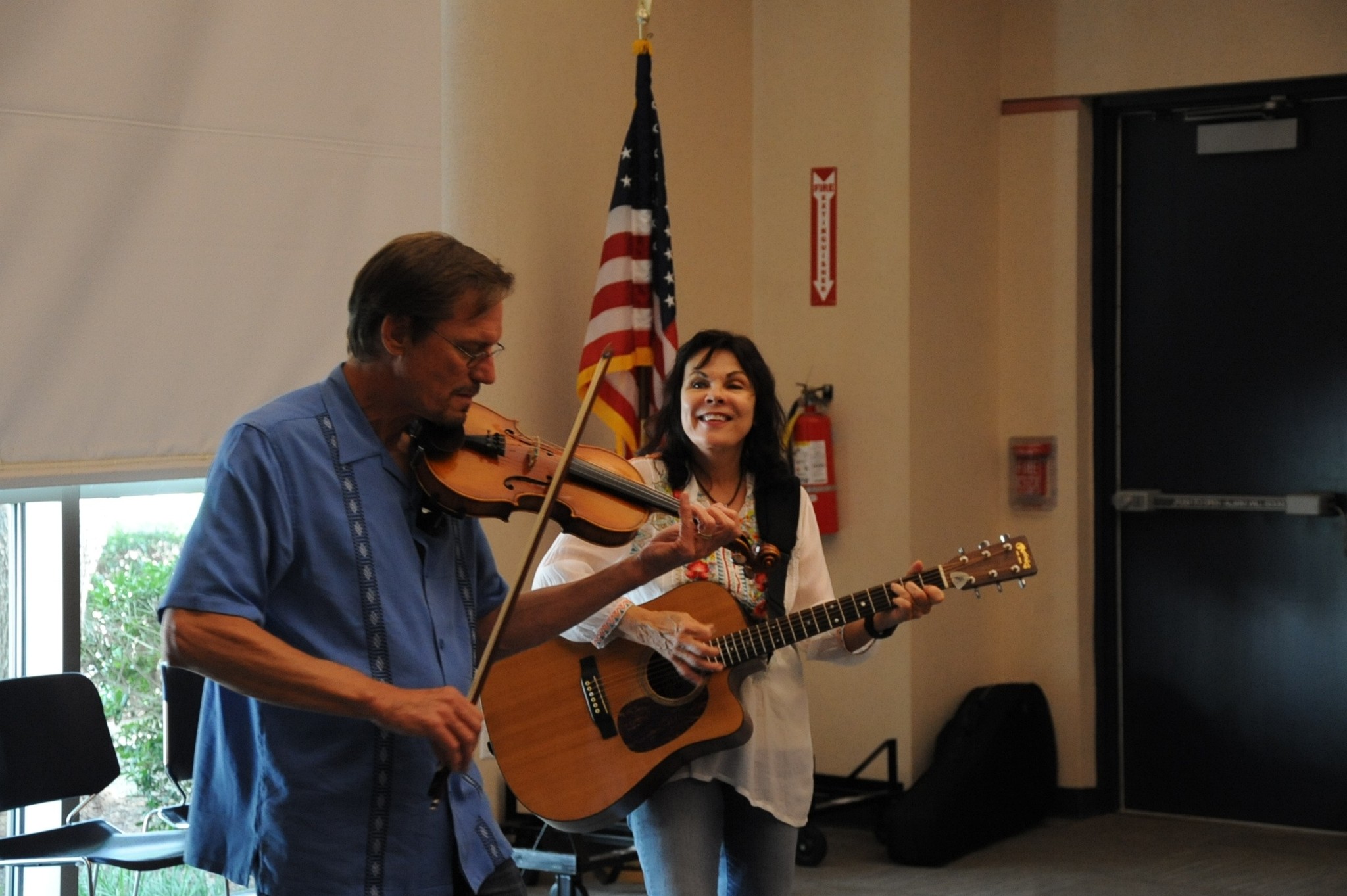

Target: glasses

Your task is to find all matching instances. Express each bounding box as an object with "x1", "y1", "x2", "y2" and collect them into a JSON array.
[{"x1": 400, "y1": 312, "x2": 506, "y2": 369}]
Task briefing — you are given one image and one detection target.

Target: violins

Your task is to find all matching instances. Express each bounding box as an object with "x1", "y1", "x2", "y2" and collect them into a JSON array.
[{"x1": 412, "y1": 400, "x2": 782, "y2": 579}]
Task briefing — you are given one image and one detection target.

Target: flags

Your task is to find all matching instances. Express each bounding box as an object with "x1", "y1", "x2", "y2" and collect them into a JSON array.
[{"x1": 577, "y1": 44, "x2": 680, "y2": 458}]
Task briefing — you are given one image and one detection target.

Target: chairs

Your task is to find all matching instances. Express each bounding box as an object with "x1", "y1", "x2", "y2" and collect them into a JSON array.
[
  {"x1": 0, "y1": 673, "x2": 187, "y2": 896},
  {"x1": 132, "y1": 662, "x2": 230, "y2": 896}
]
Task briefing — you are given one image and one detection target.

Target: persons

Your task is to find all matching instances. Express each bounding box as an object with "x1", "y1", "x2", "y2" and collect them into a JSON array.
[
  {"x1": 529, "y1": 329, "x2": 945, "y2": 896},
  {"x1": 156, "y1": 231, "x2": 740, "y2": 895}
]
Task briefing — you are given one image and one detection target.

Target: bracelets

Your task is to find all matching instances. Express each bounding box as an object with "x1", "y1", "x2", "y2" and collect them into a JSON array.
[{"x1": 863, "y1": 613, "x2": 896, "y2": 639}]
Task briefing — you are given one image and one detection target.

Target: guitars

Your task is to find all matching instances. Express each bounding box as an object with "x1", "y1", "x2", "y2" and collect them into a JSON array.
[{"x1": 480, "y1": 532, "x2": 1038, "y2": 833}]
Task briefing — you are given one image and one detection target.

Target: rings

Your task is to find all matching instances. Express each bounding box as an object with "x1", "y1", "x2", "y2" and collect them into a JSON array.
[{"x1": 697, "y1": 523, "x2": 713, "y2": 540}]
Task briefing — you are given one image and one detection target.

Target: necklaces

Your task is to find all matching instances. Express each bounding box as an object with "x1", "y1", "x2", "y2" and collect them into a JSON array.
[{"x1": 694, "y1": 473, "x2": 742, "y2": 507}]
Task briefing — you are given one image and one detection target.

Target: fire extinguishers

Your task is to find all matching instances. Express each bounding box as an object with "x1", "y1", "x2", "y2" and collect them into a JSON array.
[{"x1": 787, "y1": 381, "x2": 839, "y2": 534}]
